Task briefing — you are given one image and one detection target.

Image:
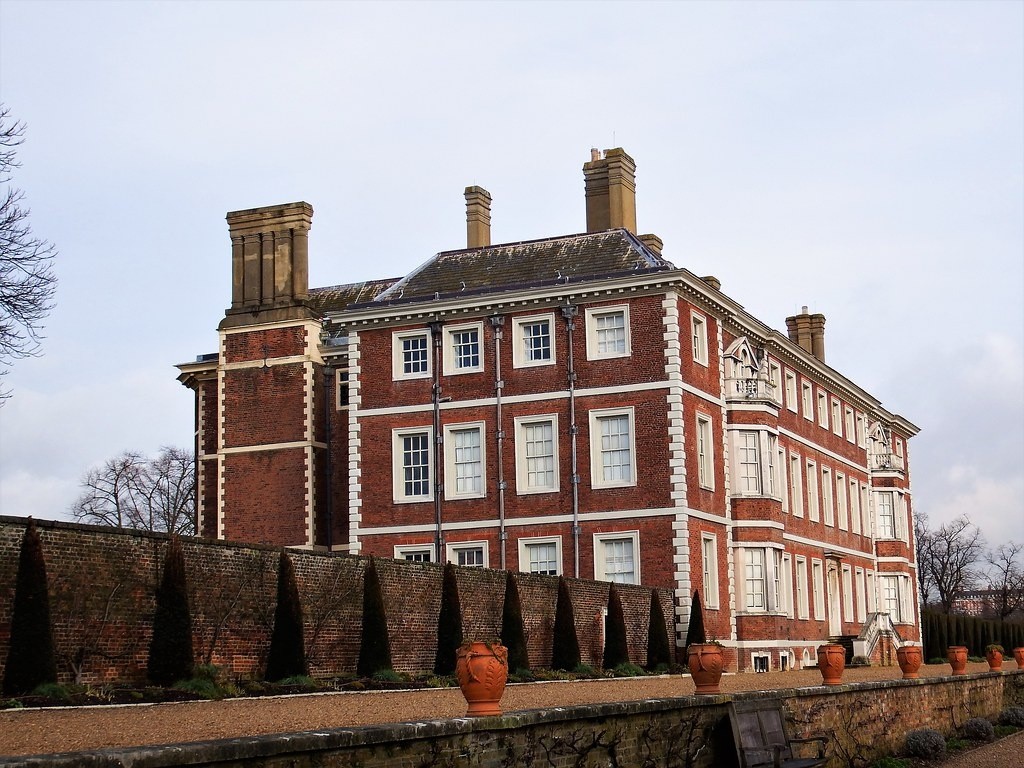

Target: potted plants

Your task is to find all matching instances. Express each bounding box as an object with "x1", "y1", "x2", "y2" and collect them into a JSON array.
[
  {"x1": 689, "y1": 641, "x2": 725, "y2": 693},
  {"x1": 897, "y1": 646, "x2": 922, "y2": 678},
  {"x1": 948, "y1": 645, "x2": 968, "y2": 674},
  {"x1": 455, "y1": 637, "x2": 508, "y2": 716},
  {"x1": 816, "y1": 644, "x2": 847, "y2": 685},
  {"x1": 1013, "y1": 646, "x2": 1024, "y2": 668},
  {"x1": 985, "y1": 644, "x2": 1004, "y2": 671}
]
